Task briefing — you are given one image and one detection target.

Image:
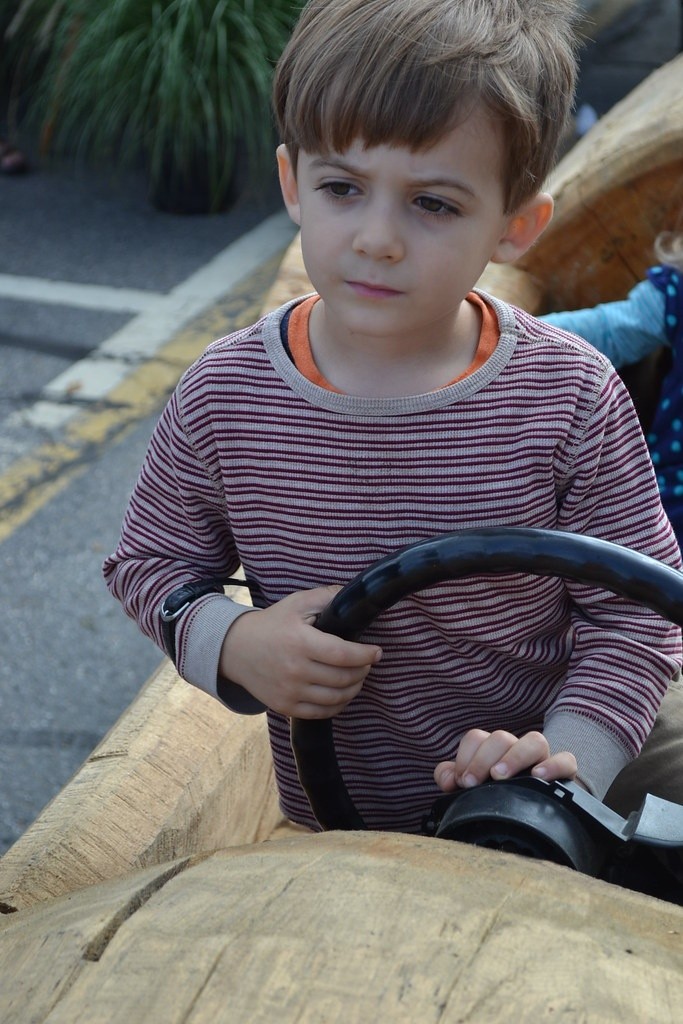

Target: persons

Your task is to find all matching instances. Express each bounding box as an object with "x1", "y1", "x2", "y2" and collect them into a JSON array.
[
  {"x1": 532, "y1": 231, "x2": 683, "y2": 563},
  {"x1": 101, "y1": 0, "x2": 683, "y2": 833}
]
